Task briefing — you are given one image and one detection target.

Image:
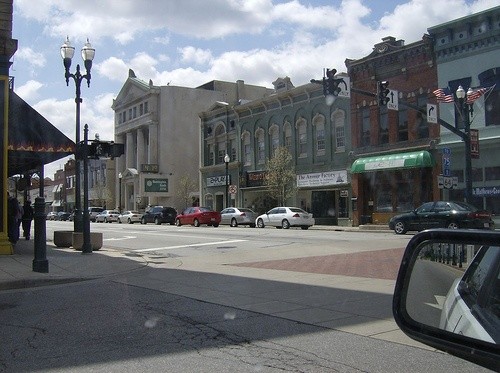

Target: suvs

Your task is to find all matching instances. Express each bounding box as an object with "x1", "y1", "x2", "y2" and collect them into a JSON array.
[
  {"x1": 141, "y1": 207, "x2": 177, "y2": 225},
  {"x1": 82, "y1": 207, "x2": 104, "y2": 222}
]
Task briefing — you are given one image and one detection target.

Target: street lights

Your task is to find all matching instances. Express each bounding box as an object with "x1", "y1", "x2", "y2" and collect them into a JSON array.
[
  {"x1": 118, "y1": 172, "x2": 122, "y2": 213},
  {"x1": 456, "y1": 86, "x2": 473, "y2": 206},
  {"x1": 60, "y1": 33, "x2": 96, "y2": 254},
  {"x1": 224, "y1": 154, "x2": 230, "y2": 208}
]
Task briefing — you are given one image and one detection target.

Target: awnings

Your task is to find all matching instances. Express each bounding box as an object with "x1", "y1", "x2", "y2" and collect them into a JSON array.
[{"x1": 350, "y1": 151, "x2": 433, "y2": 173}]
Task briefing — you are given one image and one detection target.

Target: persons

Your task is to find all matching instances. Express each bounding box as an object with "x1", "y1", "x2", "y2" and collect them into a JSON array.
[
  {"x1": 21, "y1": 200, "x2": 34, "y2": 239},
  {"x1": 7, "y1": 192, "x2": 24, "y2": 243}
]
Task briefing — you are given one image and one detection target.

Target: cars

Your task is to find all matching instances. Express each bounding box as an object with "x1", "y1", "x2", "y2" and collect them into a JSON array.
[
  {"x1": 96, "y1": 210, "x2": 121, "y2": 223},
  {"x1": 69, "y1": 212, "x2": 84, "y2": 221},
  {"x1": 219, "y1": 207, "x2": 258, "y2": 228},
  {"x1": 255, "y1": 206, "x2": 315, "y2": 230},
  {"x1": 175, "y1": 207, "x2": 221, "y2": 228},
  {"x1": 46, "y1": 212, "x2": 58, "y2": 220},
  {"x1": 389, "y1": 201, "x2": 491, "y2": 235},
  {"x1": 54, "y1": 212, "x2": 71, "y2": 221},
  {"x1": 116, "y1": 211, "x2": 145, "y2": 224}
]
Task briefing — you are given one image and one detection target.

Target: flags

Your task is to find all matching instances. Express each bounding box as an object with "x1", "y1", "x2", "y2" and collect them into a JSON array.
[
  {"x1": 467, "y1": 85, "x2": 496, "y2": 104},
  {"x1": 433, "y1": 86, "x2": 456, "y2": 103}
]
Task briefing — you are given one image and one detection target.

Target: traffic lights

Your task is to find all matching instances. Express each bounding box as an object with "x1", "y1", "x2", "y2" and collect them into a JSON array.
[
  {"x1": 377, "y1": 80, "x2": 390, "y2": 107},
  {"x1": 323, "y1": 68, "x2": 343, "y2": 96}
]
